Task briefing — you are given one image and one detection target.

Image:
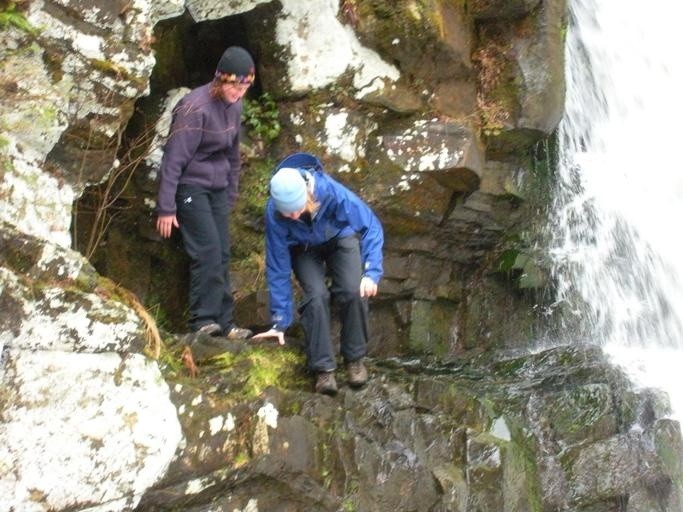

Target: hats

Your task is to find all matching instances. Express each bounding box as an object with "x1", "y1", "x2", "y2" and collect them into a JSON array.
[
  {"x1": 215, "y1": 45, "x2": 255, "y2": 84},
  {"x1": 269, "y1": 167, "x2": 308, "y2": 214}
]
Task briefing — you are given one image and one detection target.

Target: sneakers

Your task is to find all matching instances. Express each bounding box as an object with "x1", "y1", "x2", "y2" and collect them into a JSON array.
[
  {"x1": 346, "y1": 356, "x2": 368, "y2": 387},
  {"x1": 216, "y1": 328, "x2": 252, "y2": 340},
  {"x1": 315, "y1": 371, "x2": 338, "y2": 396},
  {"x1": 186, "y1": 323, "x2": 222, "y2": 345}
]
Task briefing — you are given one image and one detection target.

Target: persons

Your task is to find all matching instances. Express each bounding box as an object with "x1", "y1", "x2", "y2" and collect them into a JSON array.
[
  {"x1": 246, "y1": 152, "x2": 385, "y2": 396},
  {"x1": 155, "y1": 45, "x2": 256, "y2": 338}
]
